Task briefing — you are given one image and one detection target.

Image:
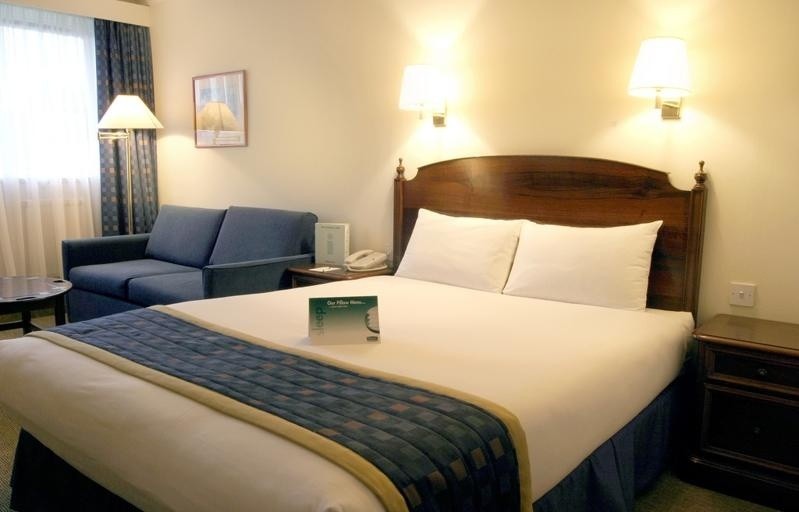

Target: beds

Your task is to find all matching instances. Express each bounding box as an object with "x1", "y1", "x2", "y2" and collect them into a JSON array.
[{"x1": 0, "y1": 158, "x2": 709, "y2": 512}]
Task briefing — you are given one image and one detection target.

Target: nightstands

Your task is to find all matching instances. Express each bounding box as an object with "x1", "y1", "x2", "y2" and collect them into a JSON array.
[
  {"x1": 690, "y1": 314, "x2": 799, "y2": 512},
  {"x1": 286, "y1": 264, "x2": 393, "y2": 287}
]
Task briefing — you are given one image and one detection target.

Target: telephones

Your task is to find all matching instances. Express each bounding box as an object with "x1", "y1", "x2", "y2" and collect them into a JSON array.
[{"x1": 344, "y1": 249, "x2": 388, "y2": 269}]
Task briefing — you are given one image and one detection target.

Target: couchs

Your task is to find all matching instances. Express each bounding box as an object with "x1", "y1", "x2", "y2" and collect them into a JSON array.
[{"x1": 62, "y1": 204, "x2": 318, "y2": 323}]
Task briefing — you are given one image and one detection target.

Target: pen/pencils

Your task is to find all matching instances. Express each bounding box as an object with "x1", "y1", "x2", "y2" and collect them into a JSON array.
[{"x1": 17, "y1": 297, "x2": 35, "y2": 300}]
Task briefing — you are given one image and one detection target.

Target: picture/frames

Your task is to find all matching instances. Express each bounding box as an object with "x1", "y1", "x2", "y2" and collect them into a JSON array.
[{"x1": 192, "y1": 69, "x2": 248, "y2": 148}]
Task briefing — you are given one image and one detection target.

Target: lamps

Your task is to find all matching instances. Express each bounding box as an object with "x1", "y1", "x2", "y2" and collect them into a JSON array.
[
  {"x1": 99, "y1": 94, "x2": 165, "y2": 234},
  {"x1": 398, "y1": 58, "x2": 471, "y2": 125},
  {"x1": 629, "y1": 35, "x2": 698, "y2": 120}
]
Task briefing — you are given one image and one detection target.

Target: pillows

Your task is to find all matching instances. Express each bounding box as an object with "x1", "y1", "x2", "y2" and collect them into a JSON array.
[
  {"x1": 395, "y1": 208, "x2": 528, "y2": 294},
  {"x1": 145, "y1": 204, "x2": 228, "y2": 268},
  {"x1": 207, "y1": 207, "x2": 317, "y2": 265},
  {"x1": 504, "y1": 220, "x2": 662, "y2": 314}
]
